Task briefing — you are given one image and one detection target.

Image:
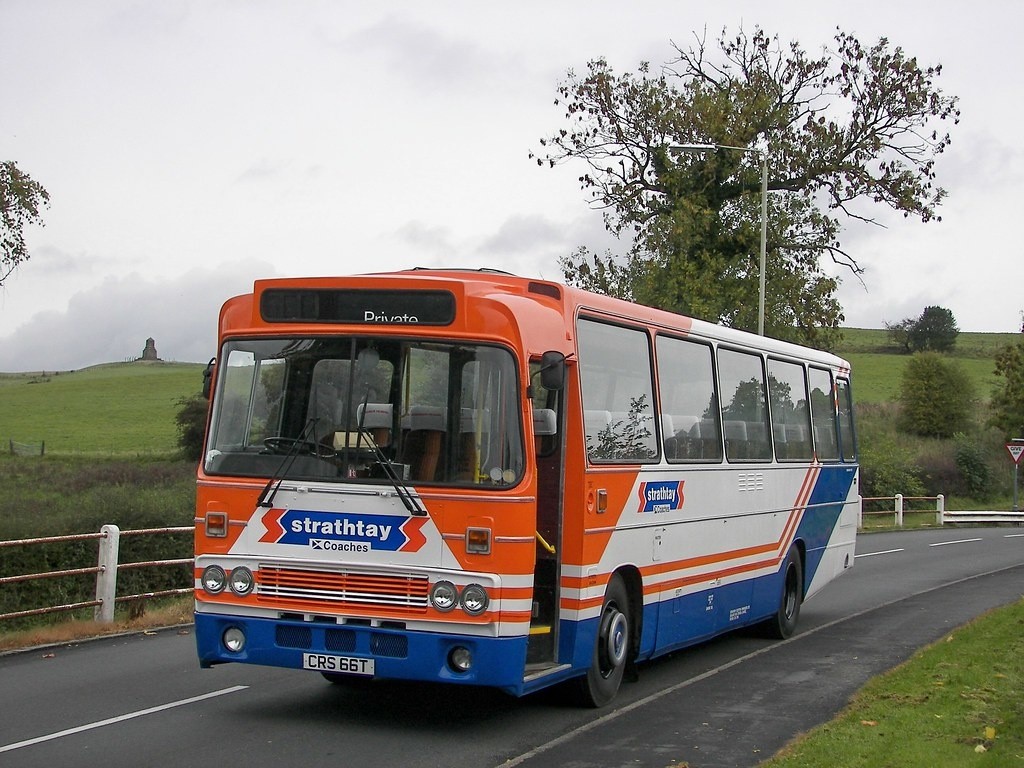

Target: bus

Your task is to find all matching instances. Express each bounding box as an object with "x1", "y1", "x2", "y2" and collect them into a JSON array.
[{"x1": 191, "y1": 270, "x2": 863, "y2": 710}]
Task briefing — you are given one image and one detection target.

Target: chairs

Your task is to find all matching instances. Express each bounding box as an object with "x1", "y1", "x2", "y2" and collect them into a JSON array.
[{"x1": 355, "y1": 398, "x2": 854, "y2": 489}]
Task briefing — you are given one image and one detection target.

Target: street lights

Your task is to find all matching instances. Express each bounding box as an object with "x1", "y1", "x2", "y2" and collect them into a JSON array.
[{"x1": 669, "y1": 140, "x2": 767, "y2": 337}]
